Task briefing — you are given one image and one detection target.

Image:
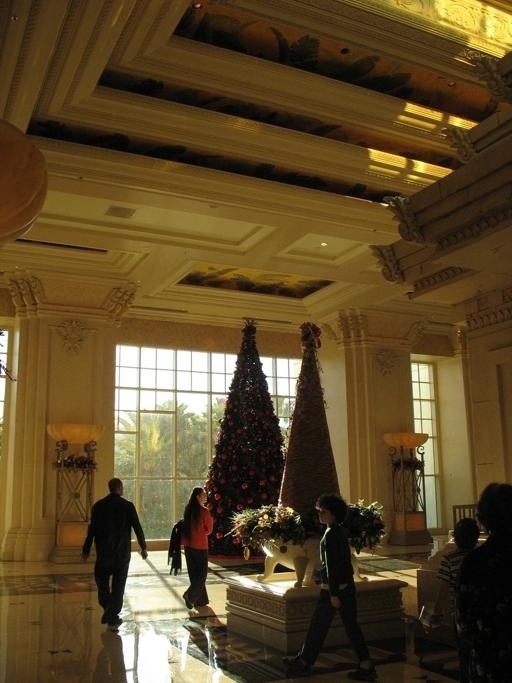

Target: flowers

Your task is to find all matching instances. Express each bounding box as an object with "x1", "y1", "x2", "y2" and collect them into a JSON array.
[{"x1": 231, "y1": 498, "x2": 390, "y2": 555}]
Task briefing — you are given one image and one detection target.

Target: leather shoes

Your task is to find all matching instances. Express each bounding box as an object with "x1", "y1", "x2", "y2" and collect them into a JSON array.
[
  {"x1": 101, "y1": 609, "x2": 123, "y2": 626},
  {"x1": 183, "y1": 592, "x2": 209, "y2": 609}
]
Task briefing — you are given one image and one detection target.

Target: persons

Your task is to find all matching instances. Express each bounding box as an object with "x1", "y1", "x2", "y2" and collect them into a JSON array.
[
  {"x1": 180, "y1": 488, "x2": 213, "y2": 609},
  {"x1": 422, "y1": 518, "x2": 479, "y2": 683},
  {"x1": 282, "y1": 493, "x2": 378, "y2": 680},
  {"x1": 80, "y1": 479, "x2": 147, "y2": 626},
  {"x1": 455, "y1": 483, "x2": 512, "y2": 683}
]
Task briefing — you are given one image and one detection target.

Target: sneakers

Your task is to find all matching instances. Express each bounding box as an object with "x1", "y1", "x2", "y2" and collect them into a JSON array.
[
  {"x1": 281, "y1": 656, "x2": 311, "y2": 676},
  {"x1": 348, "y1": 663, "x2": 378, "y2": 680}
]
